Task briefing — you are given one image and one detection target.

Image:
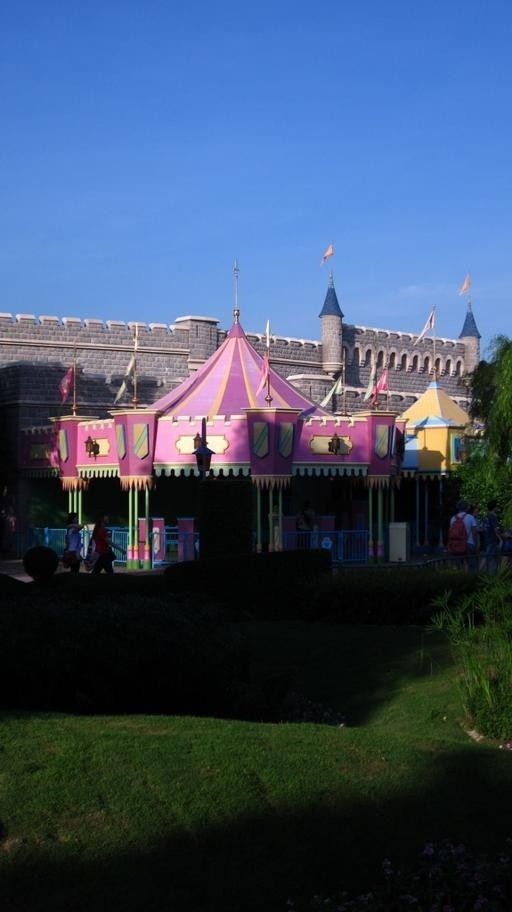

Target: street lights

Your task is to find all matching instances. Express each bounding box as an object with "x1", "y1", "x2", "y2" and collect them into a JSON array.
[{"x1": 191, "y1": 414, "x2": 217, "y2": 479}]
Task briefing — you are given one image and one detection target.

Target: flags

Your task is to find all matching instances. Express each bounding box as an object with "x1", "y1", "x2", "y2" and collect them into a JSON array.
[
  {"x1": 414, "y1": 311, "x2": 437, "y2": 346},
  {"x1": 364, "y1": 366, "x2": 376, "y2": 402},
  {"x1": 319, "y1": 244, "x2": 334, "y2": 268},
  {"x1": 58, "y1": 359, "x2": 74, "y2": 407},
  {"x1": 458, "y1": 275, "x2": 471, "y2": 297},
  {"x1": 112, "y1": 346, "x2": 139, "y2": 406},
  {"x1": 368, "y1": 368, "x2": 388, "y2": 407},
  {"x1": 320, "y1": 374, "x2": 343, "y2": 407},
  {"x1": 256, "y1": 321, "x2": 276, "y2": 397}
]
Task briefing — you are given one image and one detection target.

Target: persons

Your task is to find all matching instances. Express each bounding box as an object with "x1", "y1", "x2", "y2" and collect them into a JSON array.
[
  {"x1": 62, "y1": 513, "x2": 85, "y2": 574},
  {"x1": 450, "y1": 497, "x2": 504, "y2": 574},
  {"x1": 268, "y1": 504, "x2": 284, "y2": 552},
  {"x1": 295, "y1": 500, "x2": 316, "y2": 550},
  {"x1": 92, "y1": 513, "x2": 114, "y2": 574}
]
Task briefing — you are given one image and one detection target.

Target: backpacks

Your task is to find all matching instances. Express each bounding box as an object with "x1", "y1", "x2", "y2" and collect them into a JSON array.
[{"x1": 447, "y1": 513, "x2": 467, "y2": 554}]
